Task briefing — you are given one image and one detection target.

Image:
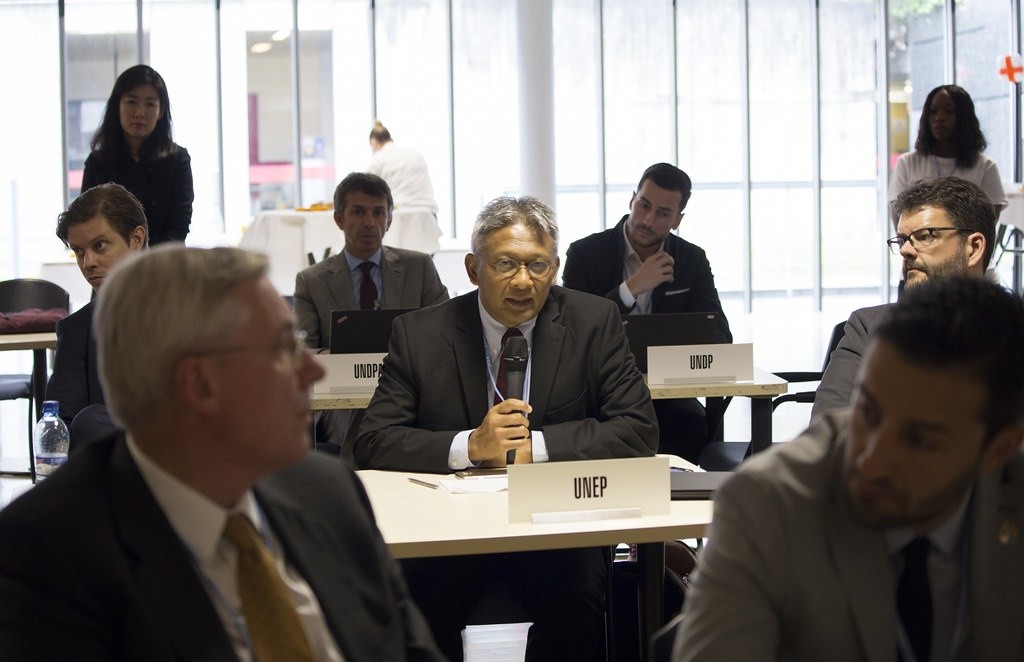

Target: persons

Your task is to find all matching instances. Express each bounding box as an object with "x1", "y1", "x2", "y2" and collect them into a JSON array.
[
  {"x1": 84, "y1": 66, "x2": 192, "y2": 245},
  {"x1": 350, "y1": 196, "x2": 659, "y2": 662},
  {"x1": 890, "y1": 84, "x2": 1008, "y2": 288},
  {"x1": 365, "y1": 122, "x2": 443, "y2": 250},
  {"x1": 45, "y1": 182, "x2": 151, "y2": 440},
  {"x1": 289, "y1": 171, "x2": 452, "y2": 446},
  {"x1": 669, "y1": 269, "x2": 1024, "y2": 662},
  {"x1": 0, "y1": 245, "x2": 448, "y2": 662},
  {"x1": 565, "y1": 163, "x2": 741, "y2": 470}
]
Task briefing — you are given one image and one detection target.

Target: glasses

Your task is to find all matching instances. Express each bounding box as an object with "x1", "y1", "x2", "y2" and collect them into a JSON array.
[
  {"x1": 473, "y1": 249, "x2": 555, "y2": 280},
  {"x1": 171, "y1": 330, "x2": 308, "y2": 382},
  {"x1": 887, "y1": 227, "x2": 975, "y2": 255}
]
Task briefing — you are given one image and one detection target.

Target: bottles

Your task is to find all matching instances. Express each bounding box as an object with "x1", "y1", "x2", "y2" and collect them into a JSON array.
[{"x1": 32, "y1": 400, "x2": 70, "y2": 487}]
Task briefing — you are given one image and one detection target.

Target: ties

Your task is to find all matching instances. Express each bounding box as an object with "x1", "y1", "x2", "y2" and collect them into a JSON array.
[
  {"x1": 224, "y1": 515, "x2": 316, "y2": 662},
  {"x1": 494, "y1": 328, "x2": 524, "y2": 407},
  {"x1": 358, "y1": 261, "x2": 378, "y2": 309},
  {"x1": 895, "y1": 537, "x2": 933, "y2": 662}
]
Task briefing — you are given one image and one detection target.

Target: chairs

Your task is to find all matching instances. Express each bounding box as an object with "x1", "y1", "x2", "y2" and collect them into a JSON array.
[
  {"x1": 0, "y1": 279, "x2": 70, "y2": 480},
  {"x1": 701, "y1": 322, "x2": 849, "y2": 474}
]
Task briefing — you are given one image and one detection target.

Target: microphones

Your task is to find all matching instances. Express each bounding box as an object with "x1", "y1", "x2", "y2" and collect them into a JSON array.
[{"x1": 504, "y1": 335, "x2": 529, "y2": 465}]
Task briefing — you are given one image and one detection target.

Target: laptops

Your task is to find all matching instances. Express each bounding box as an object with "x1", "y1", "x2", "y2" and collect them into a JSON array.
[
  {"x1": 621, "y1": 312, "x2": 717, "y2": 373},
  {"x1": 330, "y1": 308, "x2": 416, "y2": 354},
  {"x1": 670, "y1": 471, "x2": 734, "y2": 501}
]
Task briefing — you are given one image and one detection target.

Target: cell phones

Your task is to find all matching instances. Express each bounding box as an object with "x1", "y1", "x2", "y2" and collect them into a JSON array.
[{"x1": 455, "y1": 470, "x2": 508, "y2": 479}]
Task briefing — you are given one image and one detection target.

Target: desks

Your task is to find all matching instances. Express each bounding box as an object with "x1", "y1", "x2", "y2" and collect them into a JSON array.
[
  {"x1": 0, "y1": 331, "x2": 57, "y2": 426},
  {"x1": 354, "y1": 451, "x2": 717, "y2": 662},
  {"x1": 305, "y1": 356, "x2": 789, "y2": 460},
  {"x1": 241, "y1": 206, "x2": 444, "y2": 298}
]
